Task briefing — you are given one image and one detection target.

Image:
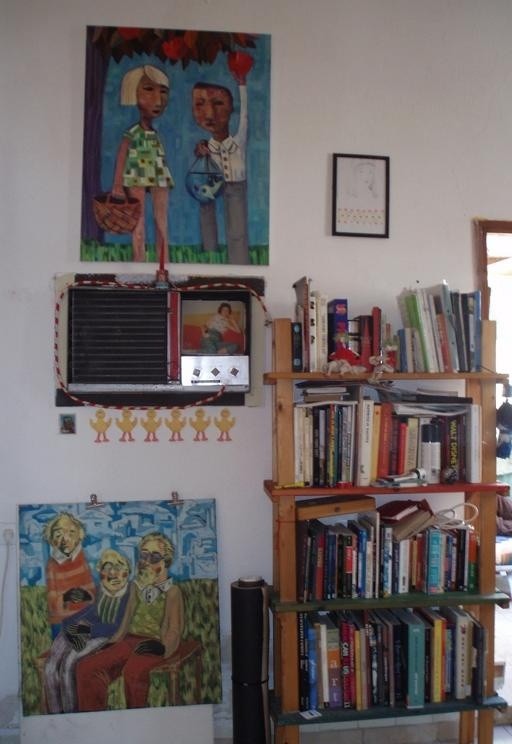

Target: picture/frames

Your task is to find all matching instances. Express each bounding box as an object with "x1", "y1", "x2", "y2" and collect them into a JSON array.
[{"x1": 330, "y1": 153, "x2": 392, "y2": 238}]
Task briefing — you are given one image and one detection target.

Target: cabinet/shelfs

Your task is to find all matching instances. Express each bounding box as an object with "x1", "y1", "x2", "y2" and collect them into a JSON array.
[{"x1": 261, "y1": 317, "x2": 511, "y2": 744}]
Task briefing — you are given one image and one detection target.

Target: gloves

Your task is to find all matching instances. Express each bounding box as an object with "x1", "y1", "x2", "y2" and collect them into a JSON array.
[
  {"x1": 64, "y1": 588, "x2": 91, "y2": 603},
  {"x1": 135, "y1": 639, "x2": 165, "y2": 655},
  {"x1": 64, "y1": 623, "x2": 91, "y2": 651}
]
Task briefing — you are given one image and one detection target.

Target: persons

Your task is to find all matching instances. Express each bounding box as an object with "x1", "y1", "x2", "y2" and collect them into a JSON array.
[
  {"x1": 113, "y1": 65, "x2": 176, "y2": 264},
  {"x1": 201, "y1": 303, "x2": 241, "y2": 354},
  {"x1": 190, "y1": 51, "x2": 256, "y2": 264},
  {"x1": 494, "y1": 493, "x2": 511, "y2": 538},
  {"x1": 41, "y1": 514, "x2": 185, "y2": 713}
]
